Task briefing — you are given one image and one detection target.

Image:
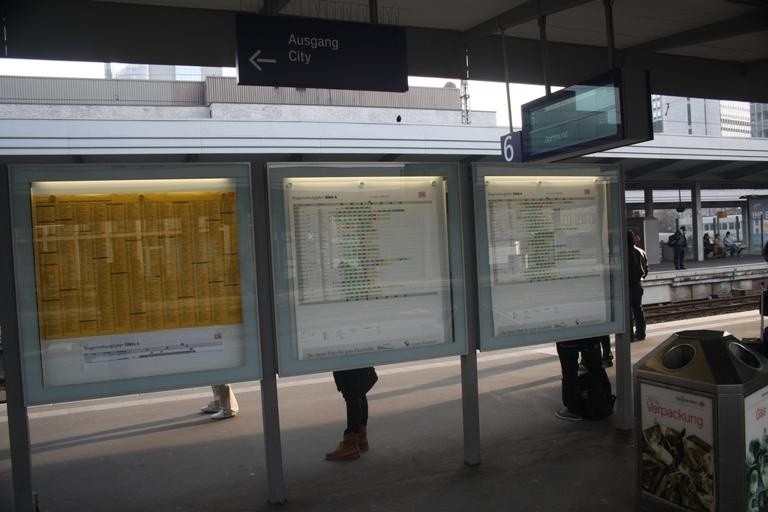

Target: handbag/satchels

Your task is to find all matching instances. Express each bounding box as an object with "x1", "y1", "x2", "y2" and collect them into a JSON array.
[
  {"x1": 669, "y1": 235, "x2": 678, "y2": 247},
  {"x1": 578, "y1": 363, "x2": 616, "y2": 420}
]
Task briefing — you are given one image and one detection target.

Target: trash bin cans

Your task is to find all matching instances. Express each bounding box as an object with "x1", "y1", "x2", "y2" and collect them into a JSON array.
[{"x1": 633, "y1": 330, "x2": 768, "y2": 512}]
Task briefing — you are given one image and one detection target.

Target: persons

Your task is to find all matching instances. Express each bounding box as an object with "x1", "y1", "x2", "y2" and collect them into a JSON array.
[
  {"x1": 199, "y1": 381, "x2": 241, "y2": 421},
  {"x1": 723, "y1": 231, "x2": 740, "y2": 256},
  {"x1": 577, "y1": 334, "x2": 614, "y2": 370},
  {"x1": 552, "y1": 333, "x2": 614, "y2": 418},
  {"x1": 668, "y1": 231, "x2": 688, "y2": 269},
  {"x1": 703, "y1": 233, "x2": 712, "y2": 257},
  {"x1": 322, "y1": 367, "x2": 378, "y2": 462},
  {"x1": 625, "y1": 228, "x2": 649, "y2": 343},
  {"x1": 714, "y1": 233, "x2": 729, "y2": 258}
]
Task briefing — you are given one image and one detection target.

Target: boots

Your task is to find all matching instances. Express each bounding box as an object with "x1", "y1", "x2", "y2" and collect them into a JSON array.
[
  {"x1": 360, "y1": 425, "x2": 369, "y2": 450},
  {"x1": 326, "y1": 429, "x2": 360, "y2": 460}
]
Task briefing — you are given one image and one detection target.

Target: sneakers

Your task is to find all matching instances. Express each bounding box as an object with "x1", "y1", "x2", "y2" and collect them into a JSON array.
[
  {"x1": 555, "y1": 407, "x2": 583, "y2": 421},
  {"x1": 211, "y1": 409, "x2": 230, "y2": 418},
  {"x1": 200, "y1": 406, "x2": 217, "y2": 412}
]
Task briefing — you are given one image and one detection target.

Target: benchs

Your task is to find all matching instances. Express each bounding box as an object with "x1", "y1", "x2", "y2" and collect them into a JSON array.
[{"x1": 711, "y1": 241, "x2": 744, "y2": 257}]
